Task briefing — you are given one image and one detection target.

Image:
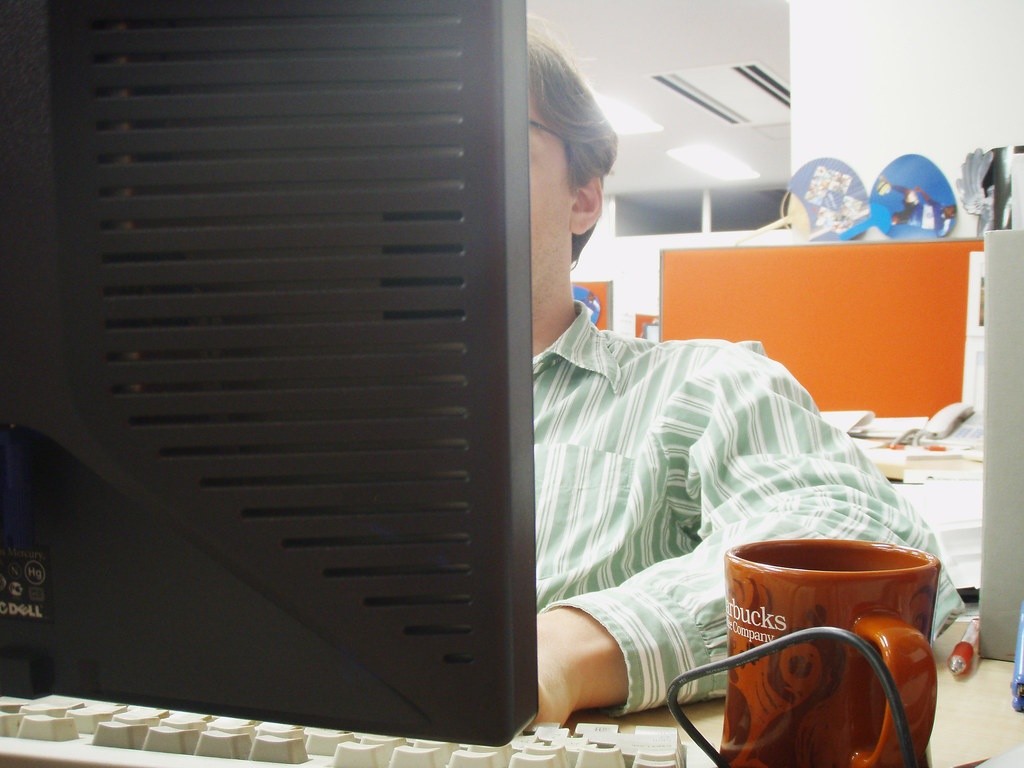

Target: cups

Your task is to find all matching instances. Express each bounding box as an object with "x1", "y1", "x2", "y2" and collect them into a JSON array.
[{"x1": 721, "y1": 540, "x2": 942, "y2": 768}]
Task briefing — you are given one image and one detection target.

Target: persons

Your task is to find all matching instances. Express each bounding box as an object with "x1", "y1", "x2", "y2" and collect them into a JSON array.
[{"x1": 529, "y1": 33, "x2": 961, "y2": 731}]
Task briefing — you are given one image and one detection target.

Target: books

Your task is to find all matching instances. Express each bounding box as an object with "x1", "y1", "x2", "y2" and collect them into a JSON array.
[{"x1": 817, "y1": 411, "x2": 964, "y2": 481}]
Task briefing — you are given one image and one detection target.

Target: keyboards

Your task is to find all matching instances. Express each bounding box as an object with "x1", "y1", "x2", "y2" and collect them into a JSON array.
[{"x1": 0, "y1": 694, "x2": 690, "y2": 768}]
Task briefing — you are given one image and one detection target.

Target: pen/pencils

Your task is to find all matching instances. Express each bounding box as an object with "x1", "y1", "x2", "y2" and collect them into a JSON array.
[
  {"x1": 924, "y1": 445, "x2": 970, "y2": 451},
  {"x1": 947, "y1": 616, "x2": 980, "y2": 676}
]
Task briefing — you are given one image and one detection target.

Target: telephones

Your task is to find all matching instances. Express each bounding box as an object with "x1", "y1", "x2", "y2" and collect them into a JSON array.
[{"x1": 917, "y1": 402, "x2": 985, "y2": 449}]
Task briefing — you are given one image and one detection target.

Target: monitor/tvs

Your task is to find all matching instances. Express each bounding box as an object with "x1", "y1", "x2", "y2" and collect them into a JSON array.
[{"x1": 0, "y1": 0, "x2": 542, "y2": 750}]
